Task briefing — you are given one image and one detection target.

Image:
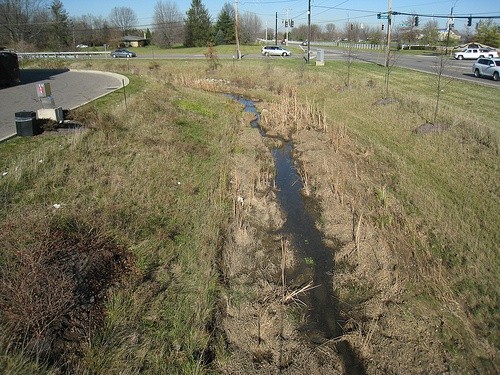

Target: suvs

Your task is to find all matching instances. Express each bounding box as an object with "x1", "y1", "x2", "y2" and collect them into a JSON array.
[
  {"x1": 472, "y1": 58, "x2": 500, "y2": 82},
  {"x1": 261, "y1": 45, "x2": 291, "y2": 57}
]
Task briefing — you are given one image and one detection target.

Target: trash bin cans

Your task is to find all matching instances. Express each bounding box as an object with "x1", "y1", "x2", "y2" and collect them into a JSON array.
[{"x1": 14, "y1": 111, "x2": 38, "y2": 137}]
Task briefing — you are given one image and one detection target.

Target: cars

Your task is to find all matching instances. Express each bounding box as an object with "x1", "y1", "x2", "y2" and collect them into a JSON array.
[
  {"x1": 110, "y1": 50, "x2": 136, "y2": 58},
  {"x1": 302, "y1": 41, "x2": 310, "y2": 46}
]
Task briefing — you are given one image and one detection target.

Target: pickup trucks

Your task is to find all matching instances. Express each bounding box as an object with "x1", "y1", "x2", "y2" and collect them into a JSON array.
[{"x1": 454, "y1": 48, "x2": 499, "y2": 59}]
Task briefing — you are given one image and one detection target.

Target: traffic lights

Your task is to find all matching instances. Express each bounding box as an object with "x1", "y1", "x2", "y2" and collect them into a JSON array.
[
  {"x1": 290, "y1": 21, "x2": 295, "y2": 27},
  {"x1": 381, "y1": 24, "x2": 384, "y2": 31},
  {"x1": 285, "y1": 22, "x2": 288, "y2": 28},
  {"x1": 351, "y1": 25, "x2": 354, "y2": 31},
  {"x1": 415, "y1": 17, "x2": 419, "y2": 27}
]
